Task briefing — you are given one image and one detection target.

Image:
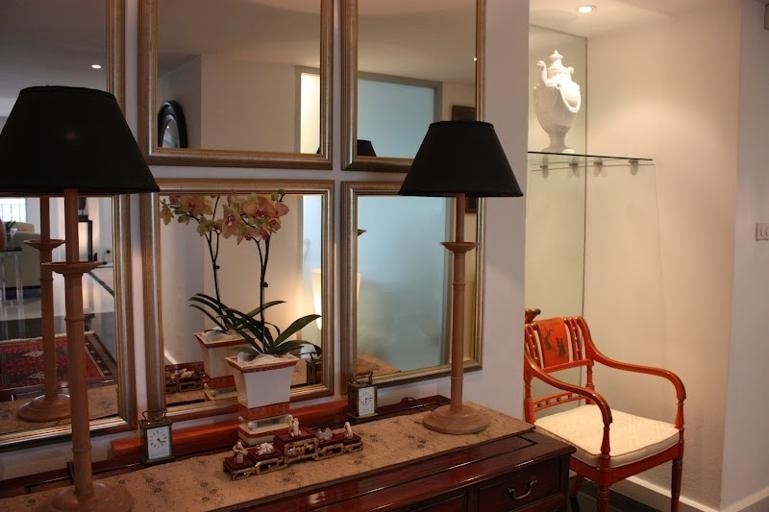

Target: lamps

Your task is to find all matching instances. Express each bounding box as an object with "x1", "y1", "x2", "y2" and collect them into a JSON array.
[
  {"x1": 0, "y1": 85, "x2": 160, "y2": 509},
  {"x1": 396, "y1": 118, "x2": 525, "y2": 436}
]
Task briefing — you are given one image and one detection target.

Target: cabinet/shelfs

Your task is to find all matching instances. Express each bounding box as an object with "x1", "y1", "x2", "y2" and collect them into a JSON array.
[{"x1": 0, "y1": 393, "x2": 583, "y2": 510}]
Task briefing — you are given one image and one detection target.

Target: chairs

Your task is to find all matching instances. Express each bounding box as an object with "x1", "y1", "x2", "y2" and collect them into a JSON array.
[{"x1": 525, "y1": 315, "x2": 687, "y2": 510}]
